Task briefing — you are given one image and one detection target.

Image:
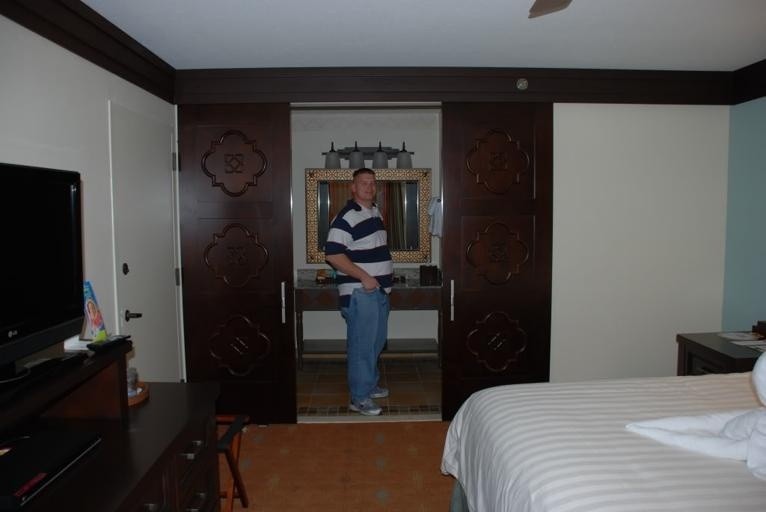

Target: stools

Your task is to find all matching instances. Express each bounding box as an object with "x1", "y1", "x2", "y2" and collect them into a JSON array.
[{"x1": 213, "y1": 415, "x2": 255, "y2": 511}]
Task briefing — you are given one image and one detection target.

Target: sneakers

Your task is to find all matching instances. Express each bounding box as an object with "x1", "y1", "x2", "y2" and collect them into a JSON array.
[
  {"x1": 367, "y1": 384, "x2": 391, "y2": 398},
  {"x1": 349, "y1": 398, "x2": 383, "y2": 416}
]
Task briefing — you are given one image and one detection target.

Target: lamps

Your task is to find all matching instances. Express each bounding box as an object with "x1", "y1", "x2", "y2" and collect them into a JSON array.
[{"x1": 318, "y1": 139, "x2": 416, "y2": 171}]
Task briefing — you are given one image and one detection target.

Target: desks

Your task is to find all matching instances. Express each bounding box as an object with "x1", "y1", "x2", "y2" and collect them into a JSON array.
[{"x1": 293, "y1": 279, "x2": 444, "y2": 370}]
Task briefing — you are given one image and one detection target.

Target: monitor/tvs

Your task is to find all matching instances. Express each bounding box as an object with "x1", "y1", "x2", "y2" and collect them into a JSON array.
[{"x1": 0, "y1": 162, "x2": 86, "y2": 381}]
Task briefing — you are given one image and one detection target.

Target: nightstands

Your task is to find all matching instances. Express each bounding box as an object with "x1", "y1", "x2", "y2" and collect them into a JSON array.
[{"x1": 673, "y1": 329, "x2": 765, "y2": 375}]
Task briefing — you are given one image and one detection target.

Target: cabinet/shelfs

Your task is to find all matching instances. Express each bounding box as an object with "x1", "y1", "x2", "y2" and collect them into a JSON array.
[{"x1": 0, "y1": 335, "x2": 224, "y2": 512}]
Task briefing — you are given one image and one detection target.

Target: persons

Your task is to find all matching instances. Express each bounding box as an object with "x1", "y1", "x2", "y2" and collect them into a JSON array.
[{"x1": 326, "y1": 170, "x2": 393, "y2": 418}]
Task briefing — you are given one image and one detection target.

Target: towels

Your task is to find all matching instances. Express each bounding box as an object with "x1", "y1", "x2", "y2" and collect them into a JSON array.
[{"x1": 426, "y1": 196, "x2": 441, "y2": 238}]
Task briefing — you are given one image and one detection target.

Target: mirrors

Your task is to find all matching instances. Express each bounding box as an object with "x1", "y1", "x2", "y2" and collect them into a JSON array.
[{"x1": 302, "y1": 167, "x2": 434, "y2": 266}]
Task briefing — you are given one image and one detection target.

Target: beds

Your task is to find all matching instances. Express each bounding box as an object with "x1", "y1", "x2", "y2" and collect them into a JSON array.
[{"x1": 439, "y1": 375, "x2": 763, "y2": 512}]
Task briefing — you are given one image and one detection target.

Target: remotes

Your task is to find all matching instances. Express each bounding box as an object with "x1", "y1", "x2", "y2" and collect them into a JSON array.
[{"x1": 86, "y1": 334, "x2": 131, "y2": 352}]
